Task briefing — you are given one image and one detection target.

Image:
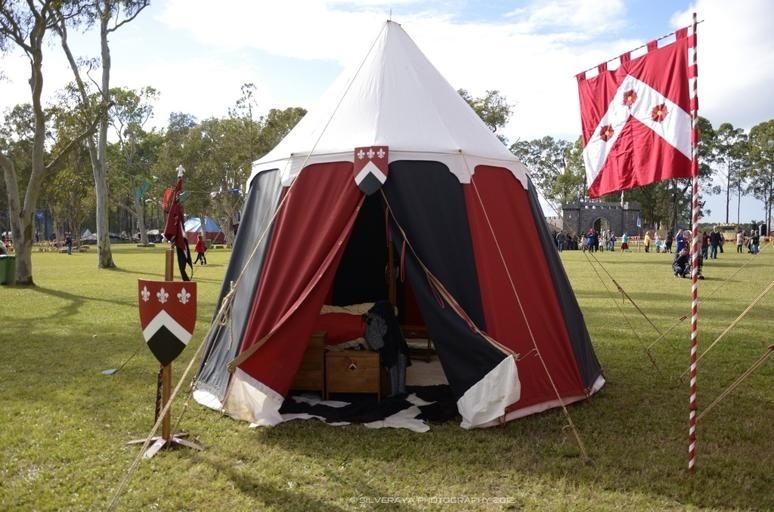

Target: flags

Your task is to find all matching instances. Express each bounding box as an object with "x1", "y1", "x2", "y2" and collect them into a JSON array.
[{"x1": 574, "y1": 23, "x2": 700, "y2": 203}]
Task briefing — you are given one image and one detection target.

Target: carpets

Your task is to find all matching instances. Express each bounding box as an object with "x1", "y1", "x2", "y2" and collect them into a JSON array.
[{"x1": 279, "y1": 358, "x2": 460, "y2": 434}]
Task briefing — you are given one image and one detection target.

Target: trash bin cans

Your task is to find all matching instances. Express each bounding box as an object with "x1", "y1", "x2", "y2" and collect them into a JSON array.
[{"x1": 0, "y1": 255, "x2": 15, "y2": 285}]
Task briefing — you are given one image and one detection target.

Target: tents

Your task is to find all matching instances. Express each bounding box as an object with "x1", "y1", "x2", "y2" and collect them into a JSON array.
[{"x1": 182, "y1": 18, "x2": 610, "y2": 434}]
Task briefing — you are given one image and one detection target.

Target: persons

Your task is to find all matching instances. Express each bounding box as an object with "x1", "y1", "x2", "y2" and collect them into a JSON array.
[
  {"x1": 551, "y1": 219, "x2": 762, "y2": 279},
  {"x1": 192, "y1": 234, "x2": 207, "y2": 266},
  {"x1": 64, "y1": 231, "x2": 73, "y2": 253}
]
